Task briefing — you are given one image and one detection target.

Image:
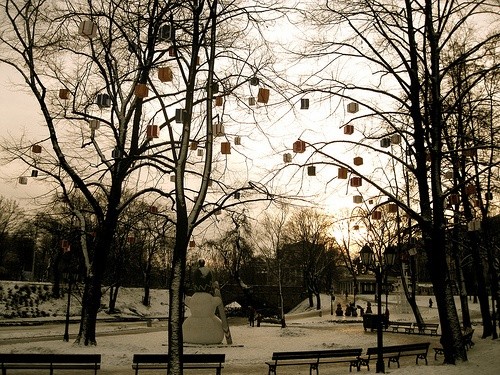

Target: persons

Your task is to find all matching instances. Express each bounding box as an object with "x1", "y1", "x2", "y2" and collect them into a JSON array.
[
  {"x1": 256, "y1": 314, "x2": 261, "y2": 327},
  {"x1": 429, "y1": 298, "x2": 433, "y2": 308},
  {"x1": 248, "y1": 307, "x2": 255, "y2": 327}
]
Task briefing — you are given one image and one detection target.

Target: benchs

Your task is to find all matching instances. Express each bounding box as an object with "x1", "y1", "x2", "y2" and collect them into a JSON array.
[
  {"x1": 132, "y1": 354, "x2": 225, "y2": 375},
  {"x1": 0, "y1": 353, "x2": 101, "y2": 375},
  {"x1": 265, "y1": 348, "x2": 362, "y2": 375},
  {"x1": 356, "y1": 321, "x2": 474, "y2": 374}
]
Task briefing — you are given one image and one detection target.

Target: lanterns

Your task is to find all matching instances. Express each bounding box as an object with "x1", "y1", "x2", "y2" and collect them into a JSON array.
[{"x1": 16, "y1": 17, "x2": 493, "y2": 233}]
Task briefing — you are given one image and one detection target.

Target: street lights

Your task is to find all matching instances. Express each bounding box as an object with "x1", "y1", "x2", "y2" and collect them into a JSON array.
[{"x1": 358, "y1": 243, "x2": 400, "y2": 374}]
